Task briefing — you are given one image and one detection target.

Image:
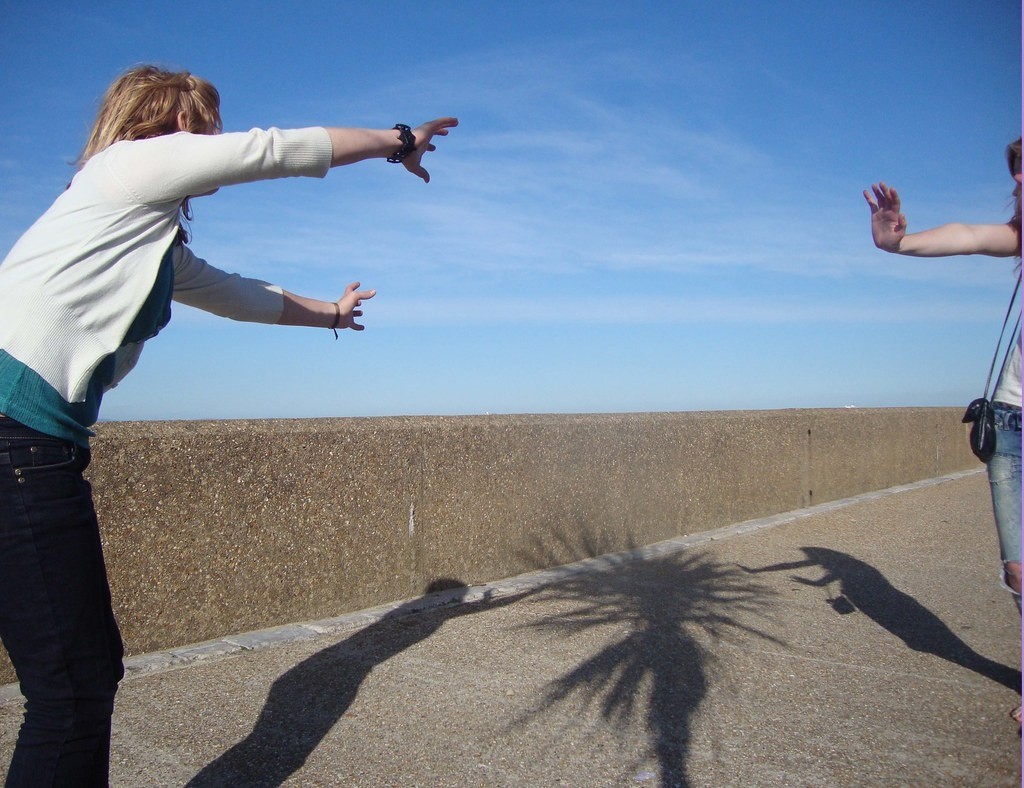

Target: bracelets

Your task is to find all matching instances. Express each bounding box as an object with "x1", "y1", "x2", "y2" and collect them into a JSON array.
[
  {"x1": 387, "y1": 124, "x2": 415, "y2": 163},
  {"x1": 328, "y1": 303, "x2": 340, "y2": 340}
]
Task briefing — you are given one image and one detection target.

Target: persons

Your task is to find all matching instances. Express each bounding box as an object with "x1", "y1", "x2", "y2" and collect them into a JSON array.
[
  {"x1": 0, "y1": 66, "x2": 459, "y2": 788},
  {"x1": 863, "y1": 137, "x2": 1022, "y2": 723}
]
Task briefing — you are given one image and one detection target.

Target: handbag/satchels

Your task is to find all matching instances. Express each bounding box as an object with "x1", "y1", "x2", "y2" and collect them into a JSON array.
[{"x1": 962, "y1": 398, "x2": 996, "y2": 462}]
